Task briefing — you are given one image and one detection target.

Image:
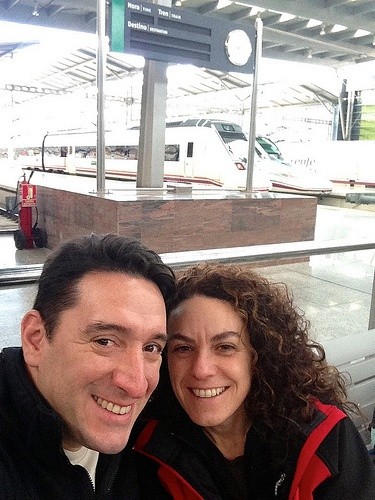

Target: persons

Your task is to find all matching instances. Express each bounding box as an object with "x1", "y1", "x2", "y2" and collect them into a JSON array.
[
  {"x1": 129, "y1": 261, "x2": 375, "y2": 499},
  {"x1": 0, "y1": 233, "x2": 181, "y2": 500}
]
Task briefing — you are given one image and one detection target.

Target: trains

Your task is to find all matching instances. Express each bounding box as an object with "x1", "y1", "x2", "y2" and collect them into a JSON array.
[
  {"x1": 243, "y1": 131, "x2": 334, "y2": 201},
  {"x1": 1, "y1": 117, "x2": 273, "y2": 194}
]
